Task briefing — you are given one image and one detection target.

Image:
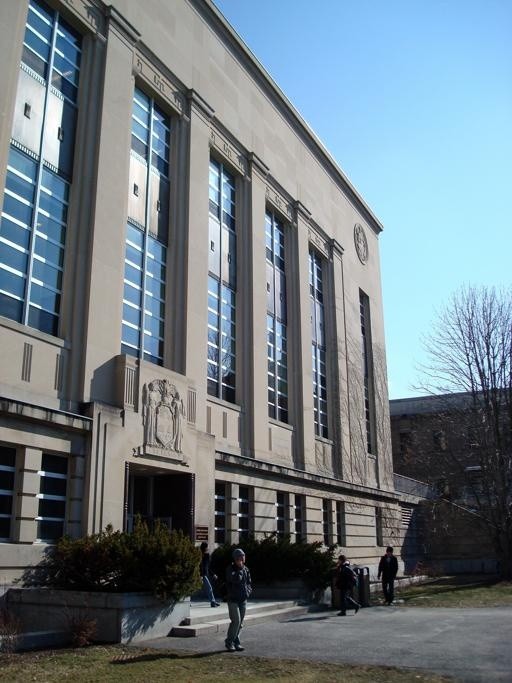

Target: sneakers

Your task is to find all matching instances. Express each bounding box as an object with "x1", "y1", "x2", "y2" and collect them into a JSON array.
[
  {"x1": 225, "y1": 641, "x2": 244, "y2": 651},
  {"x1": 211, "y1": 601, "x2": 220, "y2": 607}
]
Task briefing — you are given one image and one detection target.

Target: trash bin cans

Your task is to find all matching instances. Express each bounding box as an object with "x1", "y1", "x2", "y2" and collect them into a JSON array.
[{"x1": 332, "y1": 567, "x2": 370, "y2": 610}]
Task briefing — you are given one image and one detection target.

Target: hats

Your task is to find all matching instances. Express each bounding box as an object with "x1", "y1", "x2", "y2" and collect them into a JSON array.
[{"x1": 232, "y1": 549, "x2": 245, "y2": 558}]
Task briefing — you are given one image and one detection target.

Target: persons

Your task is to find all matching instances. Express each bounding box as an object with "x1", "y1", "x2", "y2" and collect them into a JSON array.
[
  {"x1": 224, "y1": 548, "x2": 253, "y2": 652},
  {"x1": 146, "y1": 382, "x2": 158, "y2": 448},
  {"x1": 172, "y1": 392, "x2": 184, "y2": 454},
  {"x1": 199, "y1": 542, "x2": 220, "y2": 607},
  {"x1": 377, "y1": 546, "x2": 398, "y2": 606},
  {"x1": 336, "y1": 555, "x2": 361, "y2": 616}
]
